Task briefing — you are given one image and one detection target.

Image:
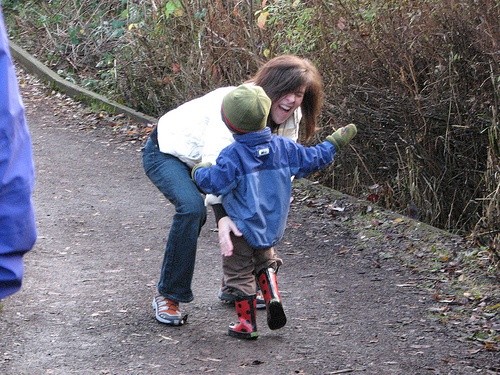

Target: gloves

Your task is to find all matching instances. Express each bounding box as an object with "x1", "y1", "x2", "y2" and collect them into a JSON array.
[
  {"x1": 191, "y1": 162, "x2": 212, "y2": 179},
  {"x1": 326, "y1": 124, "x2": 357, "y2": 152}
]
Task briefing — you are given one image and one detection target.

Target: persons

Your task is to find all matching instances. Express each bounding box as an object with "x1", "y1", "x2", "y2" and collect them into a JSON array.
[
  {"x1": 192, "y1": 85, "x2": 358, "y2": 340},
  {"x1": 142, "y1": 55, "x2": 320, "y2": 327}
]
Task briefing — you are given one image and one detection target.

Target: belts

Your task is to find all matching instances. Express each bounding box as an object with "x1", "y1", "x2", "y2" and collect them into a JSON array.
[{"x1": 150, "y1": 125, "x2": 159, "y2": 147}]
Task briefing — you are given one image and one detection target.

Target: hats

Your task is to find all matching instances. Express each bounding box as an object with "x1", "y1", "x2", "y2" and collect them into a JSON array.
[{"x1": 221, "y1": 84, "x2": 271, "y2": 133}]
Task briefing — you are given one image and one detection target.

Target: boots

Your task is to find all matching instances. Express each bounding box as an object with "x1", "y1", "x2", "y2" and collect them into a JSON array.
[
  {"x1": 228, "y1": 295, "x2": 258, "y2": 339},
  {"x1": 258, "y1": 267, "x2": 287, "y2": 330}
]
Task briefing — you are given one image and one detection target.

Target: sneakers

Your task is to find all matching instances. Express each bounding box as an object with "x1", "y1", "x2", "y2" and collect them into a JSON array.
[
  {"x1": 152, "y1": 292, "x2": 182, "y2": 324},
  {"x1": 218, "y1": 286, "x2": 266, "y2": 308}
]
